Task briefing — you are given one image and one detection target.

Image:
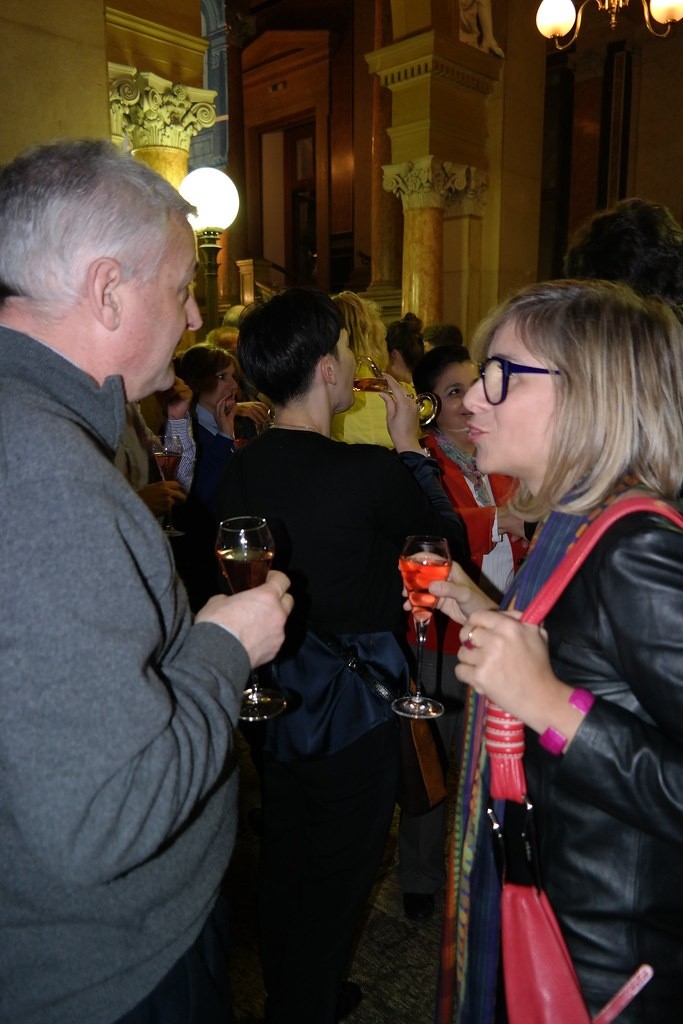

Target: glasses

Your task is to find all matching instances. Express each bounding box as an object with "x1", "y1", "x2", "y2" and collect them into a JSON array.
[{"x1": 479, "y1": 355, "x2": 565, "y2": 407}]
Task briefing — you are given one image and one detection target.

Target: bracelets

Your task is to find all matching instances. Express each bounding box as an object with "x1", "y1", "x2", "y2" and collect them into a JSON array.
[{"x1": 538, "y1": 687, "x2": 593, "y2": 758}]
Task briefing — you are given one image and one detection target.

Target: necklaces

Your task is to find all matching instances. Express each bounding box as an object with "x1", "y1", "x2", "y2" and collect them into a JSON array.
[{"x1": 272, "y1": 419, "x2": 319, "y2": 432}]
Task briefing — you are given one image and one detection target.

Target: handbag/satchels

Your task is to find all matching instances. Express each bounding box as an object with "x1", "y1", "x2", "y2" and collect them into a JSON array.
[
  {"x1": 400, "y1": 681, "x2": 448, "y2": 816},
  {"x1": 497, "y1": 850, "x2": 600, "y2": 1024}
]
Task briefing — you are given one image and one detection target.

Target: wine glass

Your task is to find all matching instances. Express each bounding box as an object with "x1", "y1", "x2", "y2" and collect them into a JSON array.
[
  {"x1": 352, "y1": 356, "x2": 442, "y2": 426},
  {"x1": 152, "y1": 435, "x2": 185, "y2": 536},
  {"x1": 215, "y1": 515, "x2": 288, "y2": 721},
  {"x1": 391, "y1": 537, "x2": 451, "y2": 718}
]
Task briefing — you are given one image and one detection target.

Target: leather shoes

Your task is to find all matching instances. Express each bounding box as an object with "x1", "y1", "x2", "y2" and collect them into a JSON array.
[
  {"x1": 337, "y1": 982, "x2": 361, "y2": 1016},
  {"x1": 402, "y1": 889, "x2": 436, "y2": 921}
]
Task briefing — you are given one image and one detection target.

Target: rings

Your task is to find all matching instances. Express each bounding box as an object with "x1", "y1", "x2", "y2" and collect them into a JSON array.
[{"x1": 466, "y1": 626, "x2": 477, "y2": 640}]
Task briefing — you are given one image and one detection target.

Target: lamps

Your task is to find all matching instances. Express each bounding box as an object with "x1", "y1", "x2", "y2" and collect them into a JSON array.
[
  {"x1": 179, "y1": 166, "x2": 239, "y2": 232},
  {"x1": 535, "y1": 0, "x2": 683, "y2": 50}
]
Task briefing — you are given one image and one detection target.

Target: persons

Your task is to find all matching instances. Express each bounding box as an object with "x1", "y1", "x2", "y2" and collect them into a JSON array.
[
  {"x1": 398, "y1": 279, "x2": 683, "y2": 1021},
  {"x1": 113, "y1": 194, "x2": 681, "y2": 1023},
  {"x1": 0, "y1": 136, "x2": 292, "y2": 1024},
  {"x1": 459, "y1": 0, "x2": 505, "y2": 59}
]
